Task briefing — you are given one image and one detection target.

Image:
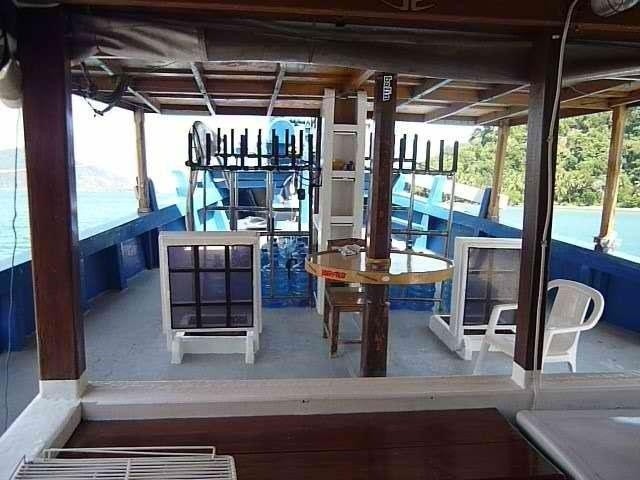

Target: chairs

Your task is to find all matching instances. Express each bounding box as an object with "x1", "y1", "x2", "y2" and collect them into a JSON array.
[
  {"x1": 324, "y1": 240, "x2": 369, "y2": 358},
  {"x1": 474, "y1": 279, "x2": 604, "y2": 377}
]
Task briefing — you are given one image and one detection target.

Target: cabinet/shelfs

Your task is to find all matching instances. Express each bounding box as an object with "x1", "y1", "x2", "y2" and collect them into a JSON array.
[{"x1": 312, "y1": 88, "x2": 366, "y2": 315}]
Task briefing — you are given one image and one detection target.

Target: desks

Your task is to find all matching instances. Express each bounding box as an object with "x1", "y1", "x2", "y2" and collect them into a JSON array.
[{"x1": 303, "y1": 248, "x2": 454, "y2": 289}]
